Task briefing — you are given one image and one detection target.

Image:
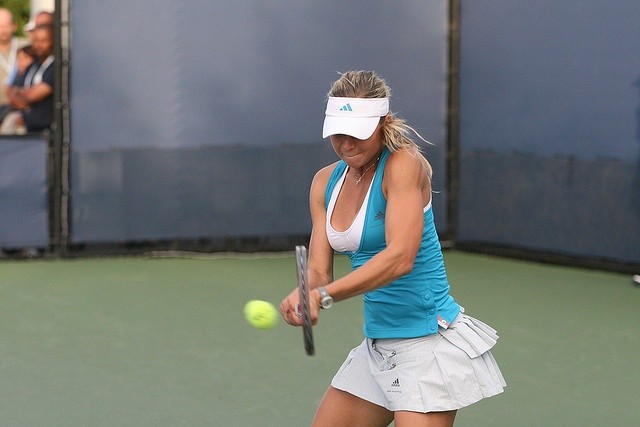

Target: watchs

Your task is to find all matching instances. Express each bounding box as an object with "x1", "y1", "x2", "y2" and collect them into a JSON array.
[{"x1": 317, "y1": 286, "x2": 334, "y2": 310}]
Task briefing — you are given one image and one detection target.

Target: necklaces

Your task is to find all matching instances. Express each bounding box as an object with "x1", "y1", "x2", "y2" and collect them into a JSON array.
[{"x1": 347, "y1": 160, "x2": 380, "y2": 185}]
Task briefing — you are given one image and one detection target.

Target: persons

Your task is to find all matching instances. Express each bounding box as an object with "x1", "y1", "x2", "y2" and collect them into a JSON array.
[
  {"x1": 277, "y1": 68, "x2": 508, "y2": 427},
  {"x1": 0, "y1": 6, "x2": 28, "y2": 82},
  {"x1": 0, "y1": 27, "x2": 57, "y2": 138},
  {"x1": 23, "y1": 10, "x2": 55, "y2": 29},
  {"x1": 0, "y1": 45, "x2": 38, "y2": 123}
]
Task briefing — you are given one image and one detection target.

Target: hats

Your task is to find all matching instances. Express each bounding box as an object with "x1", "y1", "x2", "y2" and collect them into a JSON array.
[{"x1": 322, "y1": 97, "x2": 389, "y2": 139}]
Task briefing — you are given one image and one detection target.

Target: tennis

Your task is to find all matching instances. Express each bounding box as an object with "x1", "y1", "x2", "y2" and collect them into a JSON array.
[{"x1": 243, "y1": 299, "x2": 280, "y2": 330}]
